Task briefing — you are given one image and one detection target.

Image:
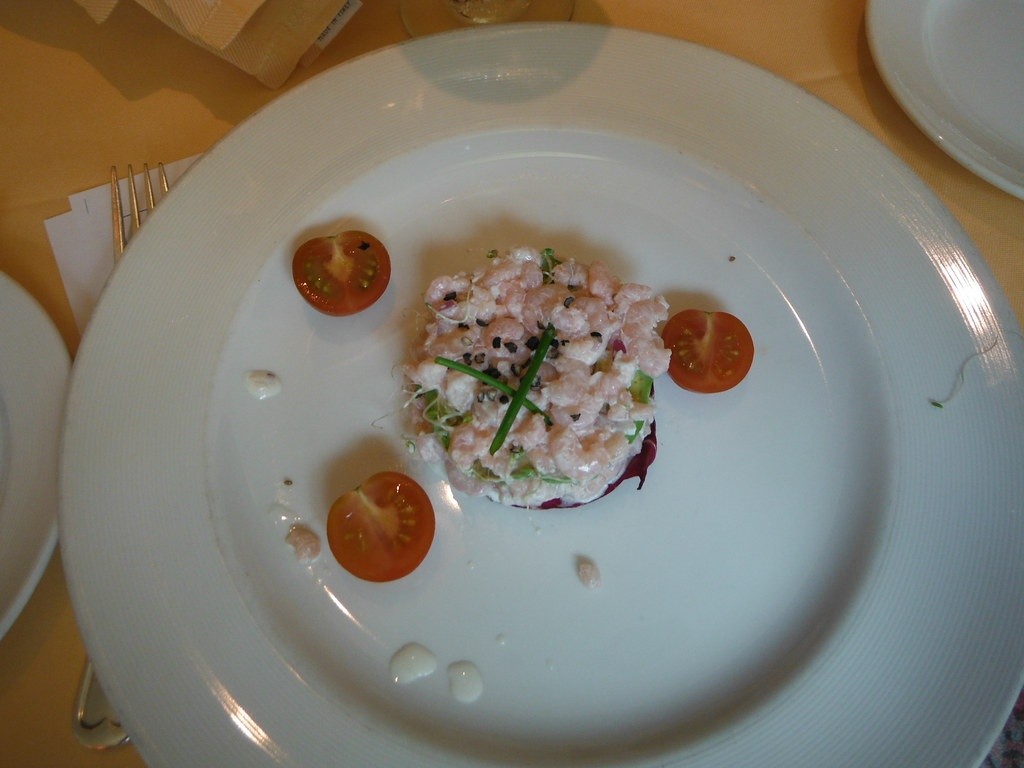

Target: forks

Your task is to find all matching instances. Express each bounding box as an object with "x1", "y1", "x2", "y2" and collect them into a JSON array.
[{"x1": 74, "y1": 158, "x2": 174, "y2": 751}]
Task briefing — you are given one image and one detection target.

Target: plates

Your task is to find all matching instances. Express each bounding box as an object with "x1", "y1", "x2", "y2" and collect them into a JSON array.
[
  {"x1": 55, "y1": 21, "x2": 1022, "y2": 767},
  {"x1": 0, "y1": 274, "x2": 74, "y2": 640},
  {"x1": 864, "y1": 2, "x2": 1022, "y2": 199}
]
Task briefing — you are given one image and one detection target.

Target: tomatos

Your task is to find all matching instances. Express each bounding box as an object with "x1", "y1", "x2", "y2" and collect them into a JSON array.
[
  {"x1": 661, "y1": 308, "x2": 757, "y2": 394},
  {"x1": 292, "y1": 232, "x2": 392, "y2": 316},
  {"x1": 326, "y1": 471, "x2": 437, "y2": 582}
]
